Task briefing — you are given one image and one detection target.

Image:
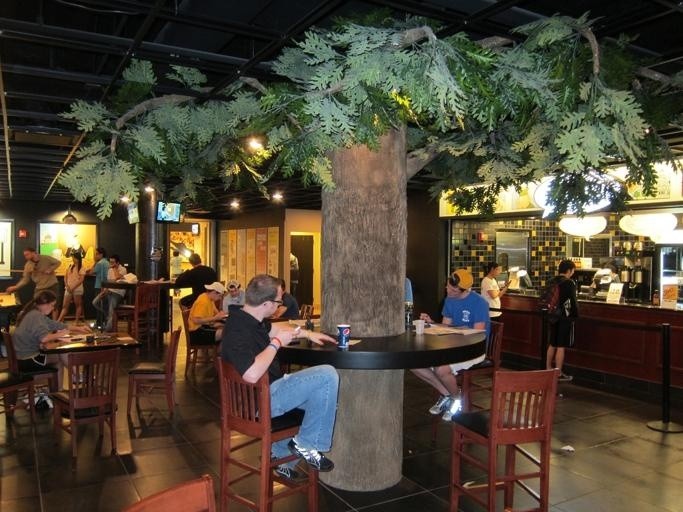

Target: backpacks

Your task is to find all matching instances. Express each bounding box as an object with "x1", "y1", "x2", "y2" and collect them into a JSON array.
[{"x1": 536, "y1": 277, "x2": 568, "y2": 324}]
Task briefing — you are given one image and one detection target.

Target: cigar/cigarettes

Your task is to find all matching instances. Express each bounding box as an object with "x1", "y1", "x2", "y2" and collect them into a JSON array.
[{"x1": 293, "y1": 326, "x2": 301, "y2": 333}]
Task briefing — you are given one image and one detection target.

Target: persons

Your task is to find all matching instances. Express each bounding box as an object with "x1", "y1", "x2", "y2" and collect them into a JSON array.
[
  {"x1": 407, "y1": 269, "x2": 490, "y2": 422},
  {"x1": 275, "y1": 280, "x2": 299, "y2": 319},
  {"x1": 6, "y1": 248, "x2": 127, "y2": 332},
  {"x1": 176, "y1": 253, "x2": 245, "y2": 346},
  {"x1": 542, "y1": 259, "x2": 579, "y2": 382},
  {"x1": 221, "y1": 275, "x2": 341, "y2": 481},
  {"x1": 481, "y1": 262, "x2": 512, "y2": 357},
  {"x1": 11, "y1": 292, "x2": 83, "y2": 392},
  {"x1": 596, "y1": 262, "x2": 620, "y2": 292}
]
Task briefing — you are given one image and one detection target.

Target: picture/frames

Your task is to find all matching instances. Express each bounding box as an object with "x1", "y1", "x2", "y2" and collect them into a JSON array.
[
  {"x1": 0, "y1": 218, "x2": 16, "y2": 281},
  {"x1": 35, "y1": 219, "x2": 103, "y2": 280}
]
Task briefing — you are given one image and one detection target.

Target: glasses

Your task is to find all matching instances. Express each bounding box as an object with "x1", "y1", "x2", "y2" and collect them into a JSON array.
[{"x1": 270, "y1": 300, "x2": 284, "y2": 306}]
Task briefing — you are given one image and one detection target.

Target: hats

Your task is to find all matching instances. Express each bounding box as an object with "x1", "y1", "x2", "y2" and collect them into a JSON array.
[
  {"x1": 226, "y1": 281, "x2": 239, "y2": 290},
  {"x1": 204, "y1": 281, "x2": 225, "y2": 294},
  {"x1": 451, "y1": 269, "x2": 473, "y2": 289}
]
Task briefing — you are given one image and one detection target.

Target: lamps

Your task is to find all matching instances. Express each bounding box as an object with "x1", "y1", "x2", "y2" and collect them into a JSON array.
[
  {"x1": 62, "y1": 201, "x2": 77, "y2": 224},
  {"x1": 618, "y1": 211, "x2": 678, "y2": 236},
  {"x1": 557, "y1": 216, "x2": 607, "y2": 238}
]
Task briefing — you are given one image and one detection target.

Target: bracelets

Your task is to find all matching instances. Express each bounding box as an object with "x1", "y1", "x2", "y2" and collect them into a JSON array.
[
  {"x1": 271, "y1": 337, "x2": 285, "y2": 349},
  {"x1": 266, "y1": 343, "x2": 280, "y2": 353}
]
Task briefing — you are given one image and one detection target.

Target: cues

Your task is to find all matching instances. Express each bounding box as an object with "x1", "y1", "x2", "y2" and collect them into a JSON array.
[{"x1": 0, "y1": 270, "x2": 61, "y2": 274}]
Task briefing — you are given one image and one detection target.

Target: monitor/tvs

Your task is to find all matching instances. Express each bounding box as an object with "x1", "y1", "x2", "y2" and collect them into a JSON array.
[{"x1": 156, "y1": 200, "x2": 181, "y2": 224}]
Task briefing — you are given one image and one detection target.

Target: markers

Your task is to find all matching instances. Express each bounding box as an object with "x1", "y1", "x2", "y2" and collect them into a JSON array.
[{"x1": 71, "y1": 337, "x2": 81, "y2": 341}]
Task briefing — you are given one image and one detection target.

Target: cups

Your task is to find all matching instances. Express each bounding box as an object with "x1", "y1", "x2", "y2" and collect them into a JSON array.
[
  {"x1": 337, "y1": 324, "x2": 351, "y2": 348},
  {"x1": 415, "y1": 320, "x2": 424, "y2": 334}
]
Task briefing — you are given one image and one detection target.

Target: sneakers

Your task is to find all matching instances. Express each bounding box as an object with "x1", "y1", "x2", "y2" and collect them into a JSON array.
[
  {"x1": 288, "y1": 437, "x2": 334, "y2": 472},
  {"x1": 271, "y1": 466, "x2": 298, "y2": 481},
  {"x1": 442, "y1": 397, "x2": 463, "y2": 421},
  {"x1": 429, "y1": 394, "x2": 450, "y2": 415},
  {"x1": 559, "y1": 373, "x2": 573, "y2": 381}
]
